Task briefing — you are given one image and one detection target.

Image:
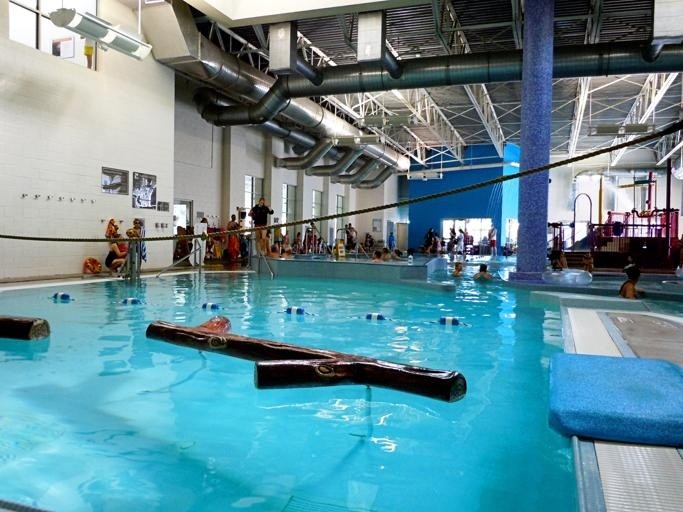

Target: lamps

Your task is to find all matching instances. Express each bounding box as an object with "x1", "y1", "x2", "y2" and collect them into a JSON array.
[
  {"x1": 586, "y1": 83, "x2": 656, "y2": 135},
  {"x1": 49, "y1": 0, "x2": 154, "y2": 61},
  {"x1": 331, "y1": 90, "x2": 444, "y2": 182}
]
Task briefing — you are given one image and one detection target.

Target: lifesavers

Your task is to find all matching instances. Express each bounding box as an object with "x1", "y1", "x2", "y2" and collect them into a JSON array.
[
  {"x1": 541, "y1": 267, "x2": 593, "y2": 286},
  {"x1": 85, "y1": 258, "x2": 102, "y2": 273}
]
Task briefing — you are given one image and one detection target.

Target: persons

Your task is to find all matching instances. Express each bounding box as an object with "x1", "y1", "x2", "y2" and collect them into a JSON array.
[
  {"x1": 248, "y1": 195, "x2": 274, "y2": 257},
  {"x1": 487, "y1": 225, "x2": 498, "y2": 255},
  {"x1": 173, "y1": 213, "x2": 250, "y2": 265},
  {"x1": 552, "y1": 250, "x2": 568, "y2": 270},
  {"x1": 123, "y1": 219, "x2": 142, "y2": 278},
  {"x1": 105, "y1": 233, "x2": 129, "y2": 273},
  {"x1": 581, "y1": 252, "x2": 593, "y2": 273},
  {"x1": 472, "y1": 264, "x2": 493, "y2": 280},
  {"x1": 620, "y1": 266, "x2": 646, "y2": 301},
  {"x1": 451, "y1": 261, "x2": 463, "y2": 277},
  {"x1": 269, "y1": 221, "x2": 465, "y2": 263},
  {"x1": 621, "y1": 254, "x2": 636, "y2": 272}
]
